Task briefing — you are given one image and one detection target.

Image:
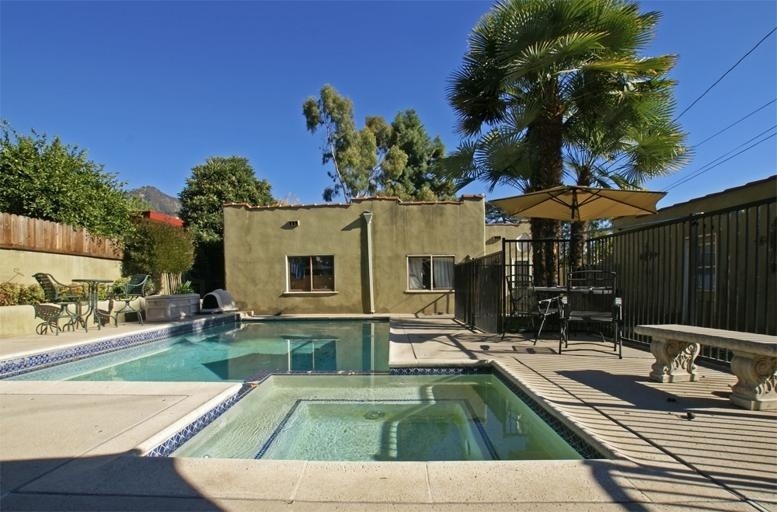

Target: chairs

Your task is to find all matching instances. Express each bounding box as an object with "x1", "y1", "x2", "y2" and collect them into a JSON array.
[
  {"x1": 501, "y1": 266, "x2": 626, "y2": 360},
  {"x1": 30, "y1": 271, "x2": 151, "y2": 337}
]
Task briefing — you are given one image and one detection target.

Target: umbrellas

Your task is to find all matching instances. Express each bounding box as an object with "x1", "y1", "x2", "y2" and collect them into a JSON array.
[{"x1": 486, "y1": 184, "x2": 669, "y2": 223}]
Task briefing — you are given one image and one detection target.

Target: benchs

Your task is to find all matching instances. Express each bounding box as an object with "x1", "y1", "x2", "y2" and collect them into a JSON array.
[{"x1": 633, "y1": 323, "x2": 777, "y2": 412}]
